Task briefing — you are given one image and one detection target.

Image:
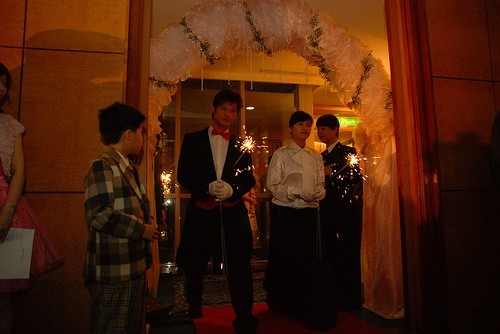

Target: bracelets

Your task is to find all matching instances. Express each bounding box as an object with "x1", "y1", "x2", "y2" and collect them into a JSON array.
[{"x1": 4, "y1": 202, "x2": 16, "y2": 208}]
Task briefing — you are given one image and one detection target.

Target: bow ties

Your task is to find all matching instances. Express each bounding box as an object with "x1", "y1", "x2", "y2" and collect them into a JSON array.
[{"x1": 211, "y1": 127, "x2": 232, "y2": 140}]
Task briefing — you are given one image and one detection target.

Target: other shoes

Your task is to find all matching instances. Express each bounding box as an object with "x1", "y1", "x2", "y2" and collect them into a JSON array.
[{"x1": 190, "y1": 305, "x2": 203, "y2": 319}]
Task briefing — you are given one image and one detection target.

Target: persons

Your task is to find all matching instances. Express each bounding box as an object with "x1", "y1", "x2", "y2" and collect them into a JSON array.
[
  {"x1": 176, "y1": 89, "x2": 363, "y2": 334},
  {"x1": 0, "y1": 61, "x2": 65, "y2": 334},
  {"x1": 82, "y1": 101, "x2": 160, "y2": 334}
]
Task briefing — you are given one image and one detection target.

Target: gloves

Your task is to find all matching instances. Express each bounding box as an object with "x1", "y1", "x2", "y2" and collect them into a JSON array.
[
  {"x1": 208, "y1": 179, "x2": 233, "y2": 202},
  {"x1": 300, "y1": 186, "x2": 326, "y2": 202}
]
